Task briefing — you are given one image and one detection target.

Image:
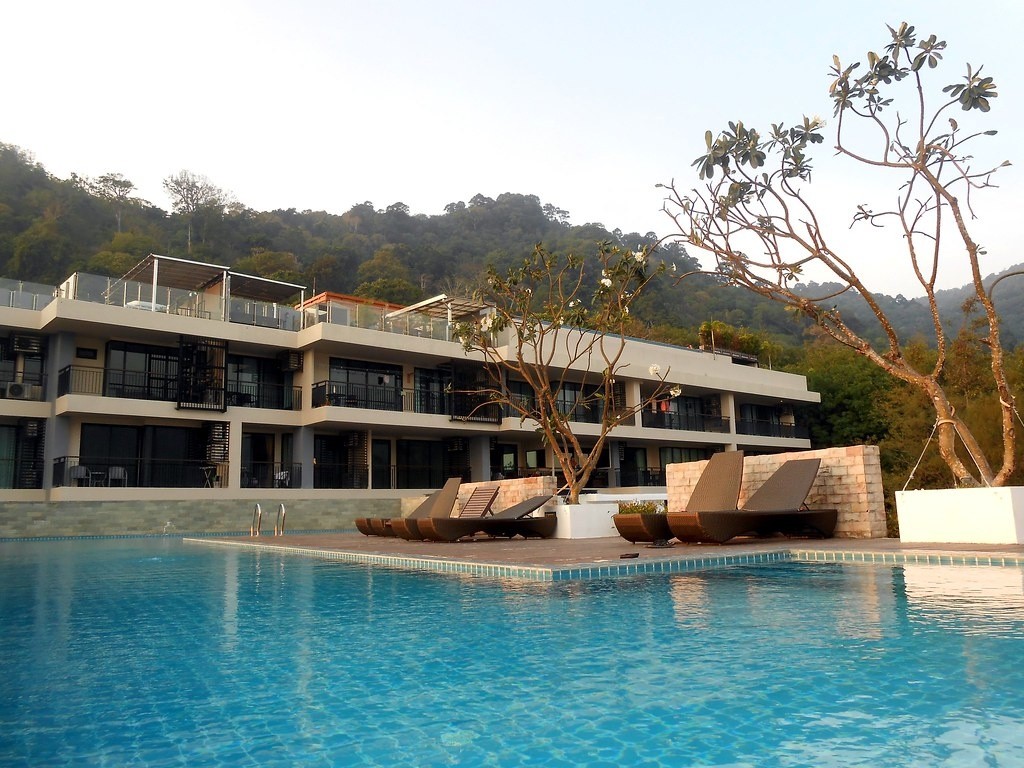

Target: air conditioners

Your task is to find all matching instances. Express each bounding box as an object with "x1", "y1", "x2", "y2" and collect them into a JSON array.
[
  {"x1": 13, "y1": 334, "x2": 40, "y2": 354},
  {"x1": 6, "y1": 381, "x2": 32, "y2": 400}
]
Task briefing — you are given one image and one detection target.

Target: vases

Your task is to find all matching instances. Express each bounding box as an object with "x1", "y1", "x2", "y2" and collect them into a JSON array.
[
  {"x1": 355, "y1": 478, "x2": 558, "y2": 544},
  {"x1": 612, "y1": 514, "x2": 675, "y2": 545}
]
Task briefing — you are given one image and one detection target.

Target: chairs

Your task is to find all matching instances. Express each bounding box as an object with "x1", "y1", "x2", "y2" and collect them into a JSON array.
[
  {"x1": 108, "y1": 467, "x2": 128, "y2": 487},
  {"x1": 70, "y1": 465, "x2": 91, "y2": 487},
  {"x1": 670, "y1": 449, "x2": 837, "y2": 547}
]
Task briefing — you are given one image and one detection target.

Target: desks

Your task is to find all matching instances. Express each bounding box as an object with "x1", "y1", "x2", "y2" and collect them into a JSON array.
[
  {"x1": 199, "y1": 466, "x2": 217, "y2": 488},
  {"x1": 92, "y1": 471, "x2": 106, "y2": 487}
]
